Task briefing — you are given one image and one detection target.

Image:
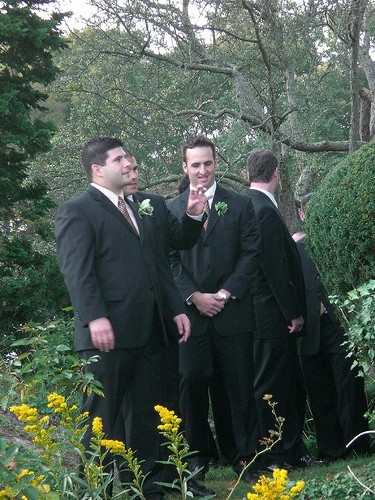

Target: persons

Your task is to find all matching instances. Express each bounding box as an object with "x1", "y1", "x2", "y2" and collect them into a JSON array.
[
  {"x1": 55, "y1": 138, "x2": 191, "y2": 500},
  {"x1": 295, "y1": 192, "x2": 375, "y2": 463},
  {"x1": 168, "y1": 137, "x2": 265, "y2": 486},
  {"x1": 120, "y1": 147, "x2": 217, "y2": 500},
  {"x1": 245, "y1": 149, "x2": 307, "y2": 474}
]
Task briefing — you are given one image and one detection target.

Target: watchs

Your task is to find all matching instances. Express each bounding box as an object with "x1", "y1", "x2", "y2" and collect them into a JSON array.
[{"x1": 217, "y1": 290, "x2": 228, "y2": 303}]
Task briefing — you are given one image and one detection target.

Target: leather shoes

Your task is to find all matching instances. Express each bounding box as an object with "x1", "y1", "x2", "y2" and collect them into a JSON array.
[
  {"x1": 259, "y1": 461, "x2": 295, "y2": 475},
  {"x1": 299, "y1": 455, "x2": 325, "y2": 466},
  {"x1": 168, "y1": 482, "x2": 215, "y2": 496}
]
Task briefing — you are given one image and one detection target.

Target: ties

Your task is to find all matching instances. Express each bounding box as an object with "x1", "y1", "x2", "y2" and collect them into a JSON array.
[
  {"x1": 204, "y1": 200, "x2": 210, "y2": 232},
  {"x1": 117, "y1": 196, "x2": 138, "y2": 236}
]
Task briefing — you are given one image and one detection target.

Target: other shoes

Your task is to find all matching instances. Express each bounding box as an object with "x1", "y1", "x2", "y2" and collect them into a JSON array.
[{"x1": 237, "y1": 468, "x2": 263, "y2": 484}]
